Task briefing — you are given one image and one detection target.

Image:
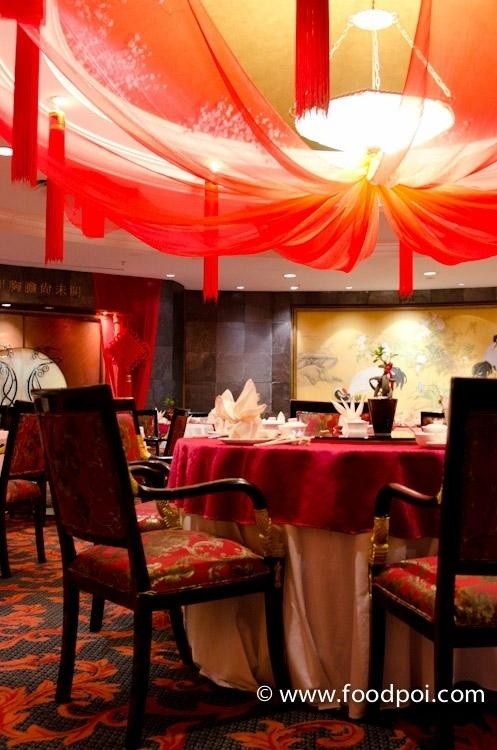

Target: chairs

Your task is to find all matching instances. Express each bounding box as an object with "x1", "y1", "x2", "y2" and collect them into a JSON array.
[
  {"x1": 366, "y1": 377, "x2": 496, "y2": 747},
  {"x1": 290, "y1": 398, "x2": 370, "y2": 438}
]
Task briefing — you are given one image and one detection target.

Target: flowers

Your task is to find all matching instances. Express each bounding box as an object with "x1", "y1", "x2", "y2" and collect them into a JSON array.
[{"x1": 367, "y1": 344, "x2": 408, "y2": 401}]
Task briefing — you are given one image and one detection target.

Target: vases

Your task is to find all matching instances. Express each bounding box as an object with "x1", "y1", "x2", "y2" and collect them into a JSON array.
[{"x1": 370, "y1": 396, "x2": 398, "y2": 432}]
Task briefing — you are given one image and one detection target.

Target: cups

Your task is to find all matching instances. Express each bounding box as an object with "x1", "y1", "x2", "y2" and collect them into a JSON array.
[
  {"x1": 368, "y1": 397, "x2": 396, "y2": 436},
  {"x1": 318, "y1": 413, "x2": 332, "y2": 432},
  {"x1": 296, "y1": 411, "x2": 316, "y2": 439}
]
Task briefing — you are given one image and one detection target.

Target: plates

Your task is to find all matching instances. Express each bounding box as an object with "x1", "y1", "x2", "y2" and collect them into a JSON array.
[
  {"x1": 218, "y1": 437, "x2": 271, "y2": 444},
  {"x1": 261, "y1": 421, "x2": 285, "y2": 425}
]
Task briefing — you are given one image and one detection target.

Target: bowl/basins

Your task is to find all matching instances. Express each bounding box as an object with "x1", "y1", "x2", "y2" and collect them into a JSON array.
[
  {"x1": 277, "y1": 422, "x2": 306, "y2": 435},
  {"x1": 348, "y1": 421, "x2": 368, "y2": 434},
  {"x1": 421, "y1": 424, "x2": 447, "y2": 435},
  {"x1": 415, "y1": 433, "x2": 445, "y2": 445}
]
